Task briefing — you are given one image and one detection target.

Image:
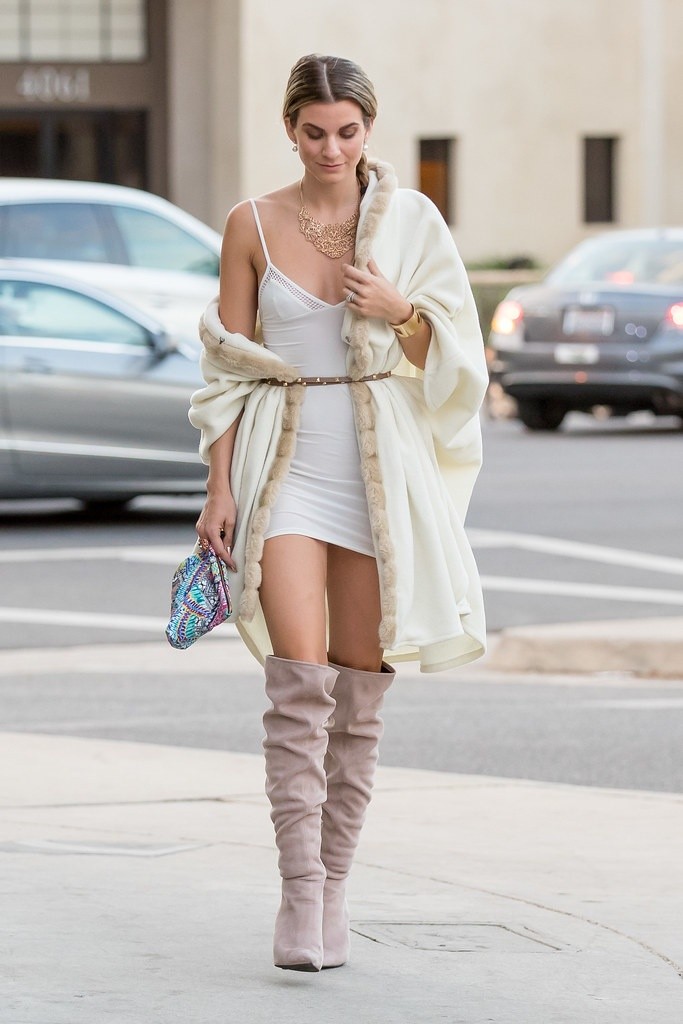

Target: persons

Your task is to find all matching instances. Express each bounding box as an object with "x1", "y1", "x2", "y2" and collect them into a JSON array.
[{"x1": 188, "y1": 52, "x2": 488, "y2": 973}]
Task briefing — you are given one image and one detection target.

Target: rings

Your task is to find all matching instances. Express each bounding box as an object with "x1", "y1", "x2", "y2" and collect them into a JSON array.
[{"x1": 346, "y1": 292, "x2": 356, "y2": 303}]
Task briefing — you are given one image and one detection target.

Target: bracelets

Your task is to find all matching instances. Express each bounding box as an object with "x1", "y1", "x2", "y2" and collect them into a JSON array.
[{"x1": 387, "y1": 302, "x2": 424, "y2": 337}]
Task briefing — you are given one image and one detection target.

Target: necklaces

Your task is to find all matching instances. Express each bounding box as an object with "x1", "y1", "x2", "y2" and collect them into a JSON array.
[{"x1": 297, "y1": 175, "x2": 362, "y2": 258}]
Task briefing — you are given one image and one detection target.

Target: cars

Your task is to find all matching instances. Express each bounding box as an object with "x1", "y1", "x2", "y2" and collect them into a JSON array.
[
  {"x1": 486, "y1": 227, "x2": 683, "y2": 431},
  {"x1": 0, "y1": 176, "x2": 224, "y2": 518}
]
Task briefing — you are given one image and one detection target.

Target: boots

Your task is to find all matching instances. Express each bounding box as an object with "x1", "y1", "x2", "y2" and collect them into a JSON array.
[
  {"x1": 261, "y1": 654, "x2": 339, "y2": 972},
  {"x1": 323, "y1": 651, "x2": 396, "y2": 969}
]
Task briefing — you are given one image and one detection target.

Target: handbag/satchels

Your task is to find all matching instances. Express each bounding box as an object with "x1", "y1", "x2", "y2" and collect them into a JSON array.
[{"x1": 164, "y1": 543, "x2": 233, "y2": 650}]
